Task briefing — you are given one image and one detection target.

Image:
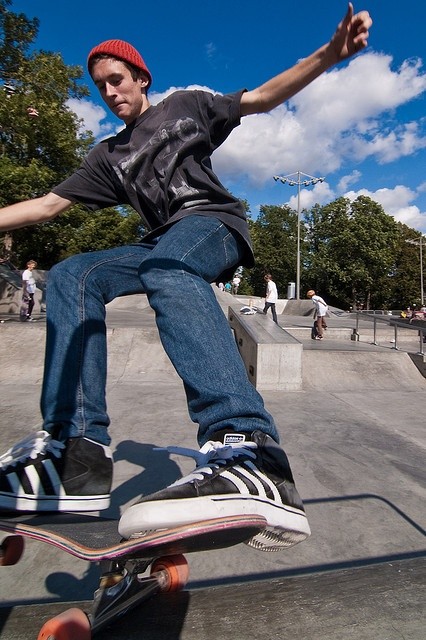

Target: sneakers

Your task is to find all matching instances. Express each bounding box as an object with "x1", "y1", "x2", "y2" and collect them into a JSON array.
[
  {"x1": 0, "y1": 431, "x2": 114, "y2": 516},
  {"x1": 119, "y1": 430, "x2": 311, "y2": 551}
]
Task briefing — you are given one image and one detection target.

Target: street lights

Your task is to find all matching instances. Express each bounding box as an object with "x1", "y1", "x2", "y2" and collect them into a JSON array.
[
  {"x1": 273, "y1": 172, "x2": 324, "y2": 298},
  {"x1": 405, "y1": 236, "x2": 423, "y2": 309}
]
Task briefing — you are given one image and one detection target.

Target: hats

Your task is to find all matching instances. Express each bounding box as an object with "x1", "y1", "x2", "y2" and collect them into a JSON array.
[{"x1": 88, "y1": 37, "x2": 152, "y2": 85}]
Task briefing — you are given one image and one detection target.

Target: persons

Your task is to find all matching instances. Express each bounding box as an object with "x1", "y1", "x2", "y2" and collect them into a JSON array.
[
  {"x1": 262, "y1": 272, "x2": 279, "y2": 325},
  {"x1": 307, "y1": 288, "x2": 331, "y2": 340},
  {"x1": 232, "y1": 273, "x2": 240, "y2": 295},
  {"x1": 22, "y1": 257, "x2": 39, "y2": 320},
  {"x1": 0, "y1": 3, "x2": 372, "y2": 552}
]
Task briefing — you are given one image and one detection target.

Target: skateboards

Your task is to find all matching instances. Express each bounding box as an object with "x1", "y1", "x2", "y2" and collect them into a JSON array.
[{"x1": 0, "y1": 508, "x2": 268, "y2": 640}]
